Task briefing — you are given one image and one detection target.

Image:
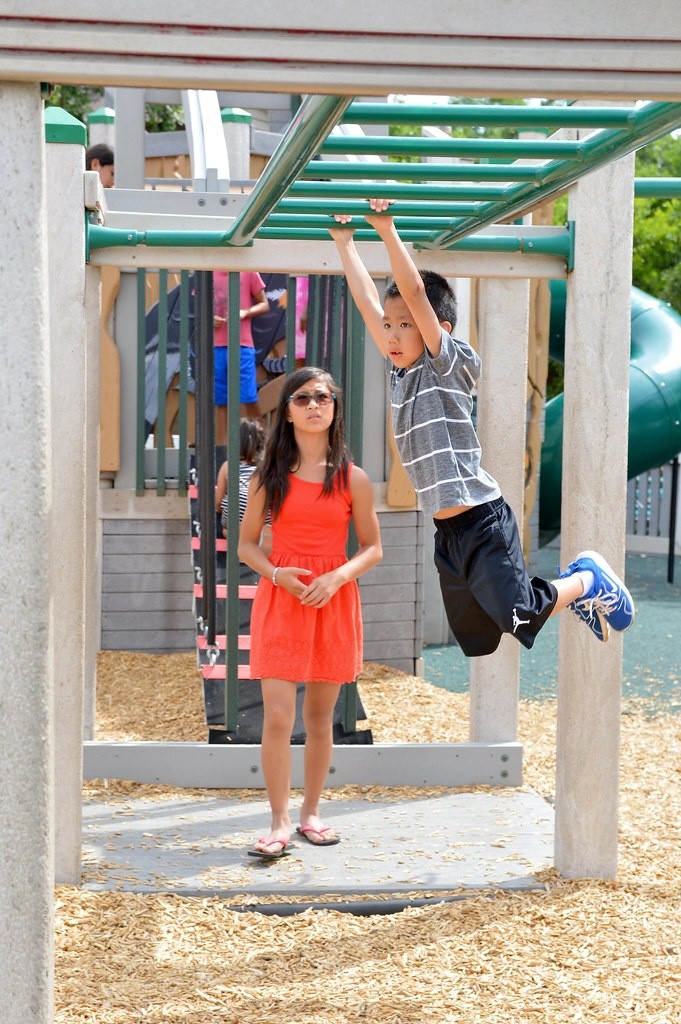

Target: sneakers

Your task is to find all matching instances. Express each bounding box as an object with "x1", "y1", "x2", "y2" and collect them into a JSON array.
[
  {"x1": 555, "y1": 566, "x2": 610, "y2": 643},
  {"x1": 569, "y1": 551, "x2": 634, "y2": 632}
]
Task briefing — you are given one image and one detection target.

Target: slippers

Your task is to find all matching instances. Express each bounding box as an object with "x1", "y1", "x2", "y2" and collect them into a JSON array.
[
  {"x1": 296, "y1": 826, "x2": 340, "y2": 845},
  {"x1": 247, "y1": 837, "x2": 288, "y2": 858}
]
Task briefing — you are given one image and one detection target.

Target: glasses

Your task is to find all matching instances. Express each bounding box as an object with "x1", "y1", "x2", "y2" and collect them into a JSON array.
[{"x1": 284, "y1": 392, "x2": 338, "y2": 407}]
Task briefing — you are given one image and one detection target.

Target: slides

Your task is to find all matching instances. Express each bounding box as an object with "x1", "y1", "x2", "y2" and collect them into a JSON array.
[{"x1": 540, "y1": 277, "x2": 681, "y2": 551}]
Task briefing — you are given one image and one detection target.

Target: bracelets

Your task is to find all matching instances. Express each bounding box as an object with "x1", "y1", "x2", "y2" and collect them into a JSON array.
[{"x1": 272, "y1": 567, "x2": 281, "y2": 586}]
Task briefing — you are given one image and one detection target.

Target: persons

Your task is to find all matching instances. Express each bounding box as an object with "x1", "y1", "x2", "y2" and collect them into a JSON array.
[
  {"x1": 235, "y1": 366, "x2": 384, "y2": 860},
  {"x1": 214, "y1": 418, "x2": 273, "y2": 558},
  {"x1": 85, "y1": 144, "x2": 114, "y2": 188},
  {"x1": 328, "y1": 198, "x2": 636, "y2": 656},
  {"x1": 192, "y1": 271, "x2": 269, "y2": 446}
]
